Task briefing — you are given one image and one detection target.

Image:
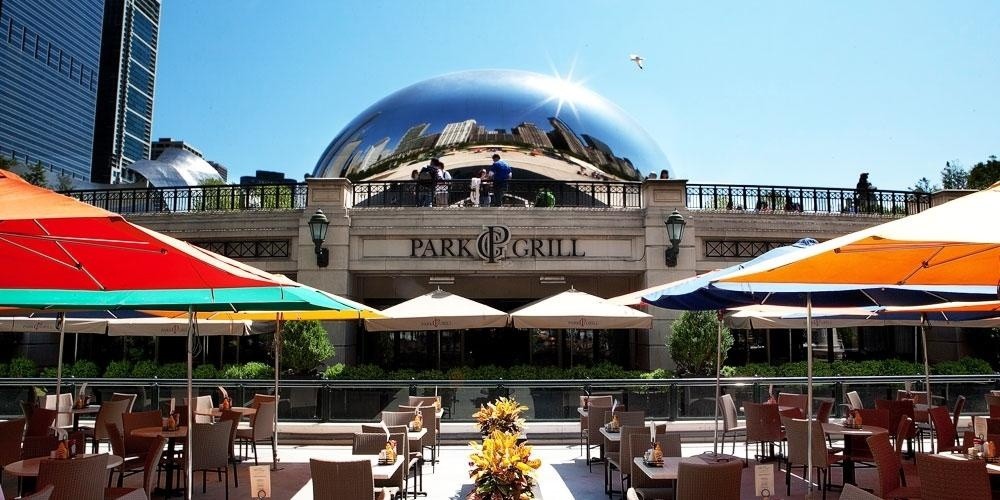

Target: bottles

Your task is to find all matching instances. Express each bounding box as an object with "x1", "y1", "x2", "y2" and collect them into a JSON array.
[
  {"x1": 161, "y1": 412, "x2": 179, "y2": 431},
  {"x1": 432, "y1": 397, "x2": 441, "y2": 413},
  {"x1": 409, "y1": 416, "x2": 422, "y2": 432},
  {"x1": 605, "y1": 416, "x2": 619, "y2": 432},
  {"x1": 847, "y1": 411, "x2": 863, "y2": 429},
  {"x1": 56, "y1": 439, "x2": 70, "y2": 460},
  {"x1": 378, "y1": 440, "x2": 397, "y2": 464},
  {"x1": 967, "y1": 440, "x2": 996, "y2": 462},
  {"x1": 77, "y1": 392, "x2": 85, "y2": 409},
  {"x1": 644, "y1": 444, "x2": 663, "y2": 462},
  {"x1": 583, "y1": 398, "x2": 588, "y2": 410},
  {"x1": 219, "y1": 394, "x2": 232, "y2": 412}
]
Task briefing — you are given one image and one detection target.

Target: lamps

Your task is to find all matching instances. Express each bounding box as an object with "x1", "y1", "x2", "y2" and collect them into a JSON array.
[
  {"x1": 662, "y1": 207, "x2": 689, "y2": 268},
  {"x1": 309, "y1": 207, "x2": 331, "y2": 269}
]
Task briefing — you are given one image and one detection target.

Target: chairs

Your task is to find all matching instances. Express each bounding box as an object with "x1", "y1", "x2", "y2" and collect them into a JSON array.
[
  {"x1": 0, "y1": 384, "x2": 280, "y2": 500},
  {"x1": 352, "y1": 396, "x2": 444, "y2": 500},
  {"x1": 718, "y1": 384, "x2": 1000, "y2": 500},
  {"x1": 310, "y1": 454, "x2": 398, "y2": 499},
  {"x1": 634, "y1": 456, "x2": 743, "y2": 499},
  {"x1": 577, "y1": 395, "x2": 681, "y2": 500}
]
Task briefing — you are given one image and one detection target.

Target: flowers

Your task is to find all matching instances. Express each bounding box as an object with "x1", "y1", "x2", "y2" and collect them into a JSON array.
[
  {"x1": 464, "y1": 429, "x2": 540, "y2": 500},
  {"x1": 469, "y1": 397, "x2": 531, "y2": 435}
]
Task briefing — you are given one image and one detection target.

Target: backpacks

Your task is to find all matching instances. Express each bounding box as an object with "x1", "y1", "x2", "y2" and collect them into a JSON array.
[{"x1": 418, "y1": 166, "x2": 435, "y2": 187}]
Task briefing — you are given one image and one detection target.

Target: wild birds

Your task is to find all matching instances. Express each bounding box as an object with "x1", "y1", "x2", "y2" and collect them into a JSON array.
[{"x1": 630, "y1": 56, "x2": 644, "y2": 69}]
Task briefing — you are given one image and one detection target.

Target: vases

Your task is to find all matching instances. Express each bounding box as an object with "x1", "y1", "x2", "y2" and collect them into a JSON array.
[
  {"x1": 479, "y1": 433, "x2": 528, "y2": 448},
  {"x1": 464, "y1": 489, "x2": 540, "y2": 499}
]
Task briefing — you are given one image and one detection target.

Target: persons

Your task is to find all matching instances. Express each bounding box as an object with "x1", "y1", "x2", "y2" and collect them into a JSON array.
[{"x1": 409, "y1": 154, "x2": 512, "y2": 207}]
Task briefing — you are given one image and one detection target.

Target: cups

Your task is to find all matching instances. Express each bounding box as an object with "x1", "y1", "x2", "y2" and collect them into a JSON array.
[{"x1": 50, "y1": 451, "x2": 55, "y2": 458}]
